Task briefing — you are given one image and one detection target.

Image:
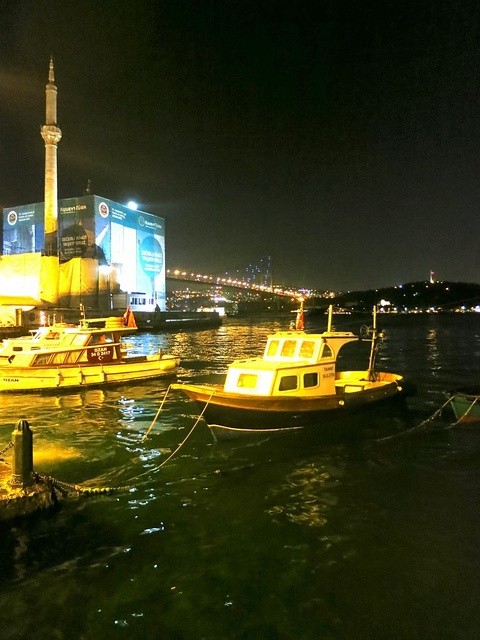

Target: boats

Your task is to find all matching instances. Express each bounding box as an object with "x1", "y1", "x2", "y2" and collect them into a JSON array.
[
  {"x1": 0, "y1": 304, "x2": 182, "y2": 394},
  {"x1": 441, "y1": 386, "x2": 479, "y2": 425},
  {"x1": 168, "y1": 302, "x2": 404, "y2": 444}
]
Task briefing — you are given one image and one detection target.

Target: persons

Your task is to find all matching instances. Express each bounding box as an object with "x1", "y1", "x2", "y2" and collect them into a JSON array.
[{"x1": 95, "y1": 333, "x2": 106, "y2": 344}]
